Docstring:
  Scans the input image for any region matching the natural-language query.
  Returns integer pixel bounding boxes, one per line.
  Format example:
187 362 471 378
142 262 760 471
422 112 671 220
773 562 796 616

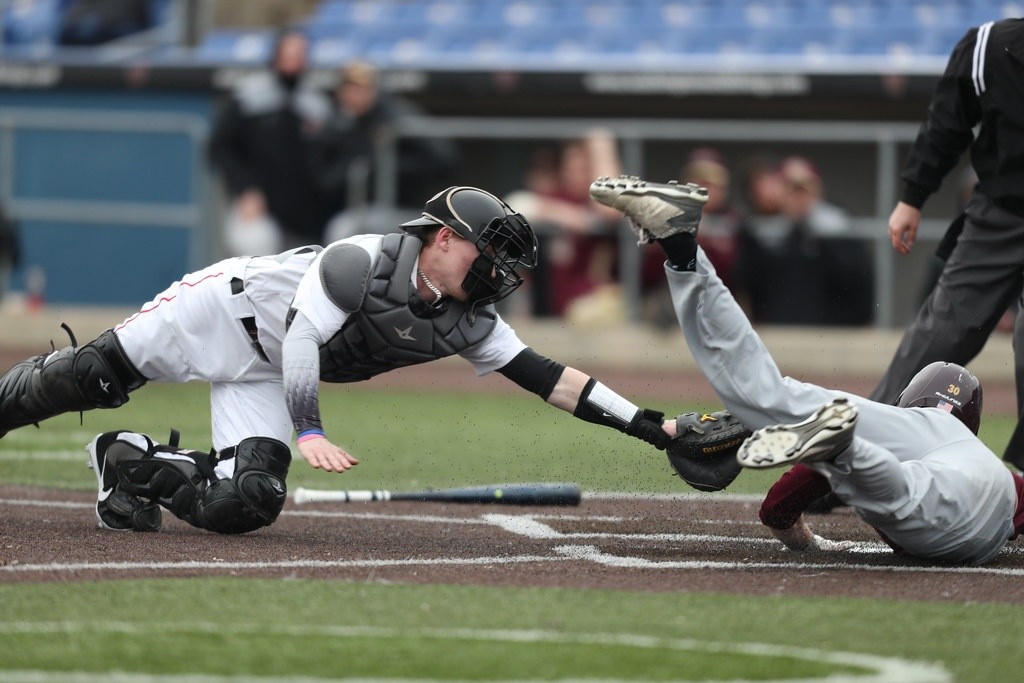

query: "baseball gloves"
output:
663 408 757 496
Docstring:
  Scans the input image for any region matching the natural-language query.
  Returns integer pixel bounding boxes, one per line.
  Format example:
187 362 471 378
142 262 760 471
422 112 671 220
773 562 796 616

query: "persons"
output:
198 30 380 255
592 174 1024 557
492 130 873 325
2 185 747 540
868 17 1023 471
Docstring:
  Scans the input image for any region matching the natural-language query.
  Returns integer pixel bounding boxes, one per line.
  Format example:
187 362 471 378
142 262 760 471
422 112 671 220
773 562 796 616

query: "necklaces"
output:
418 268 446 308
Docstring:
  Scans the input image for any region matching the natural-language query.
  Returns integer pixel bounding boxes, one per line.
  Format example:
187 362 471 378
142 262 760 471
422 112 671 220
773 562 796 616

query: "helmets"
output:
898 362 983 440
400 185 538 271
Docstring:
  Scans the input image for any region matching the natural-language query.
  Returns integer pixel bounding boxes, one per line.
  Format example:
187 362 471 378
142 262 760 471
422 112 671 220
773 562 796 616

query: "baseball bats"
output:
295 479 583 508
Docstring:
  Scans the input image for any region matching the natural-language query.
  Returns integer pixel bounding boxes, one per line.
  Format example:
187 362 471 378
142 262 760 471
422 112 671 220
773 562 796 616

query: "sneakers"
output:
738 398 859 470
590 175 710 243
86 430 143 532
